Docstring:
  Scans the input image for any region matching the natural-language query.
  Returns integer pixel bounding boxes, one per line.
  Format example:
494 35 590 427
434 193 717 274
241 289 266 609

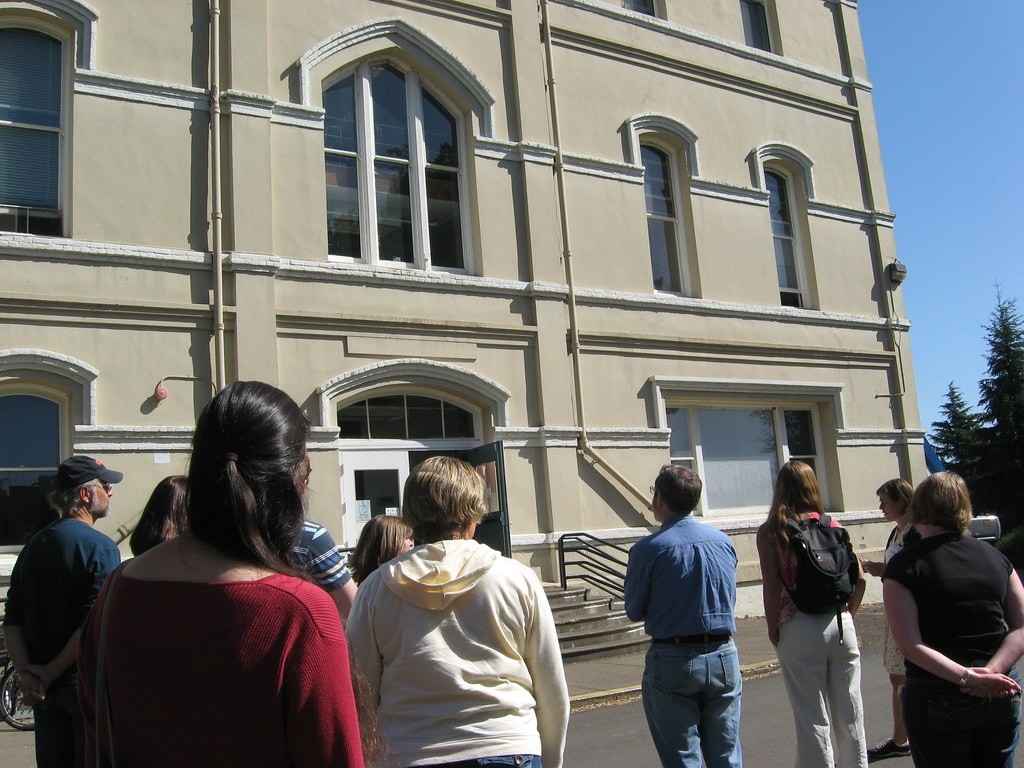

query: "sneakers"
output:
868 737 911 757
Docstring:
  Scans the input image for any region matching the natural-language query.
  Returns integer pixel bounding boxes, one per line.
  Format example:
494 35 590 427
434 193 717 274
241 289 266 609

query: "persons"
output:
856 477 916 758
349 514 415 588
2 454 125 767
76 381 369 768
343 456 571 768
879 471 1024 768
755 460 868 768
624 465 744 768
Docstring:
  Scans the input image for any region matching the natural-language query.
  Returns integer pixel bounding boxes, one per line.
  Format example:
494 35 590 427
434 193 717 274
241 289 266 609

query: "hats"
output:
58 456 123 488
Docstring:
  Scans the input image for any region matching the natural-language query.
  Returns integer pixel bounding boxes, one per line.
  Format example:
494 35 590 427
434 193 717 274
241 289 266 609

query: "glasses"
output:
90 485 112 493
649 486 658 494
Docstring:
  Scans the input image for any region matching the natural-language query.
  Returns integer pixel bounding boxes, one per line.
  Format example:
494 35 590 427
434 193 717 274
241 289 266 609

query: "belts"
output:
653 634 729 644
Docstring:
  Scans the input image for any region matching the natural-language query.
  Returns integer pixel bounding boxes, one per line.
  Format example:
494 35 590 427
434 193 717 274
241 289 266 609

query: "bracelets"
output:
958 670 975 688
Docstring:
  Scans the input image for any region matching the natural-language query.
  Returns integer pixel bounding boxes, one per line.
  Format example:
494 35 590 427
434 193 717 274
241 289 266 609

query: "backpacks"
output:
779 514 860 644
